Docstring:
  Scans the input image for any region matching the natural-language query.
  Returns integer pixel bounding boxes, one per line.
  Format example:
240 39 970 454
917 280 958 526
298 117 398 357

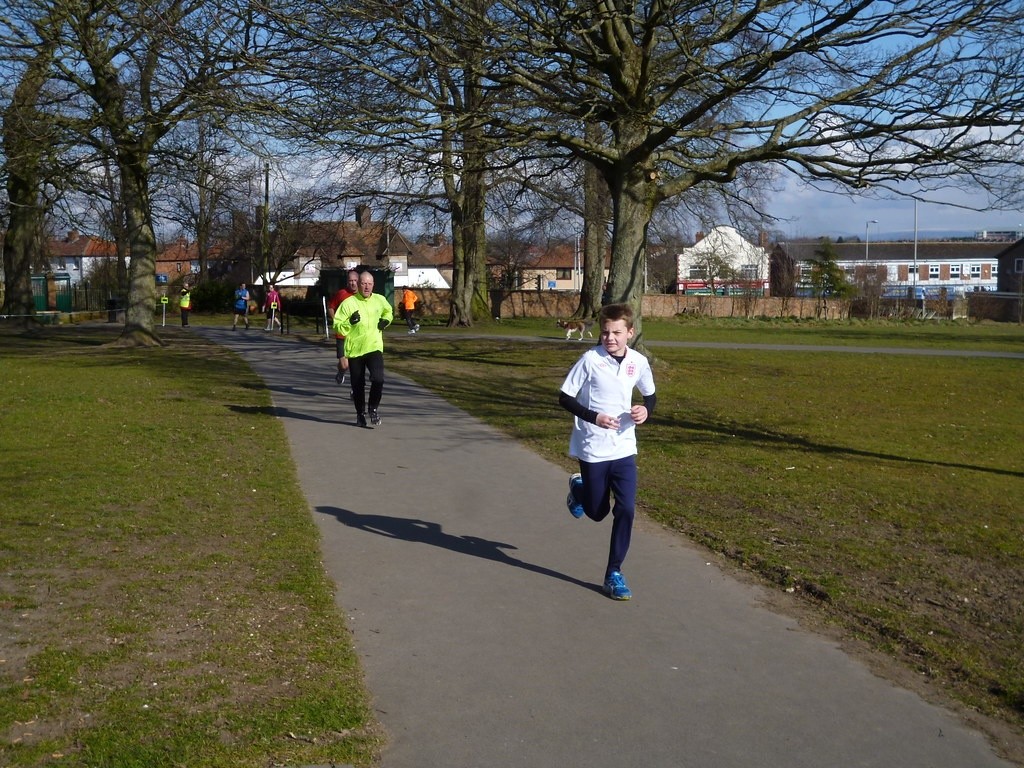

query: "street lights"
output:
865 220 879 266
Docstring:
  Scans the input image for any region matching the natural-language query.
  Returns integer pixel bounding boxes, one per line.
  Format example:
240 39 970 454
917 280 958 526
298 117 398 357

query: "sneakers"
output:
355 412 367 428
566 472 584 518
369 409 382 425
602 571 633 600
335 372 346 383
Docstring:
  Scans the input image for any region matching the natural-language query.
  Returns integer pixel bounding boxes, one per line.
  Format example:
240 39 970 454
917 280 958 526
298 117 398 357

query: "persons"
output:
179 283 192 327
263 283 282 331
231 282 250 331
333 271 393 427
401 286 420 334
328 270 358 399
559 303 657 601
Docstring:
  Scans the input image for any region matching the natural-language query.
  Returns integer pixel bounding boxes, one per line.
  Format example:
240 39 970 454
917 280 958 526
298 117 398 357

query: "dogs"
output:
556 319 592 341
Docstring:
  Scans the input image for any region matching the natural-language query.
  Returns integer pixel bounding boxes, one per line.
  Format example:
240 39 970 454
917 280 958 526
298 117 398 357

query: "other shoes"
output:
232 325 236 331
414 324 420 332
408 329 415 334
244 326 251 332
264 326 271 331
278 324 284 330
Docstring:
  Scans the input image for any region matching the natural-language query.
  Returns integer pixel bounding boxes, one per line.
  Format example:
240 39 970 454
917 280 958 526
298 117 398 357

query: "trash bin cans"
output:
106 299 126 324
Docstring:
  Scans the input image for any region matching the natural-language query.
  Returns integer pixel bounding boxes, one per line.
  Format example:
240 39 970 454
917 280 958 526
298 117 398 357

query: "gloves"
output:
350 310 360 325
378 318 390 331
